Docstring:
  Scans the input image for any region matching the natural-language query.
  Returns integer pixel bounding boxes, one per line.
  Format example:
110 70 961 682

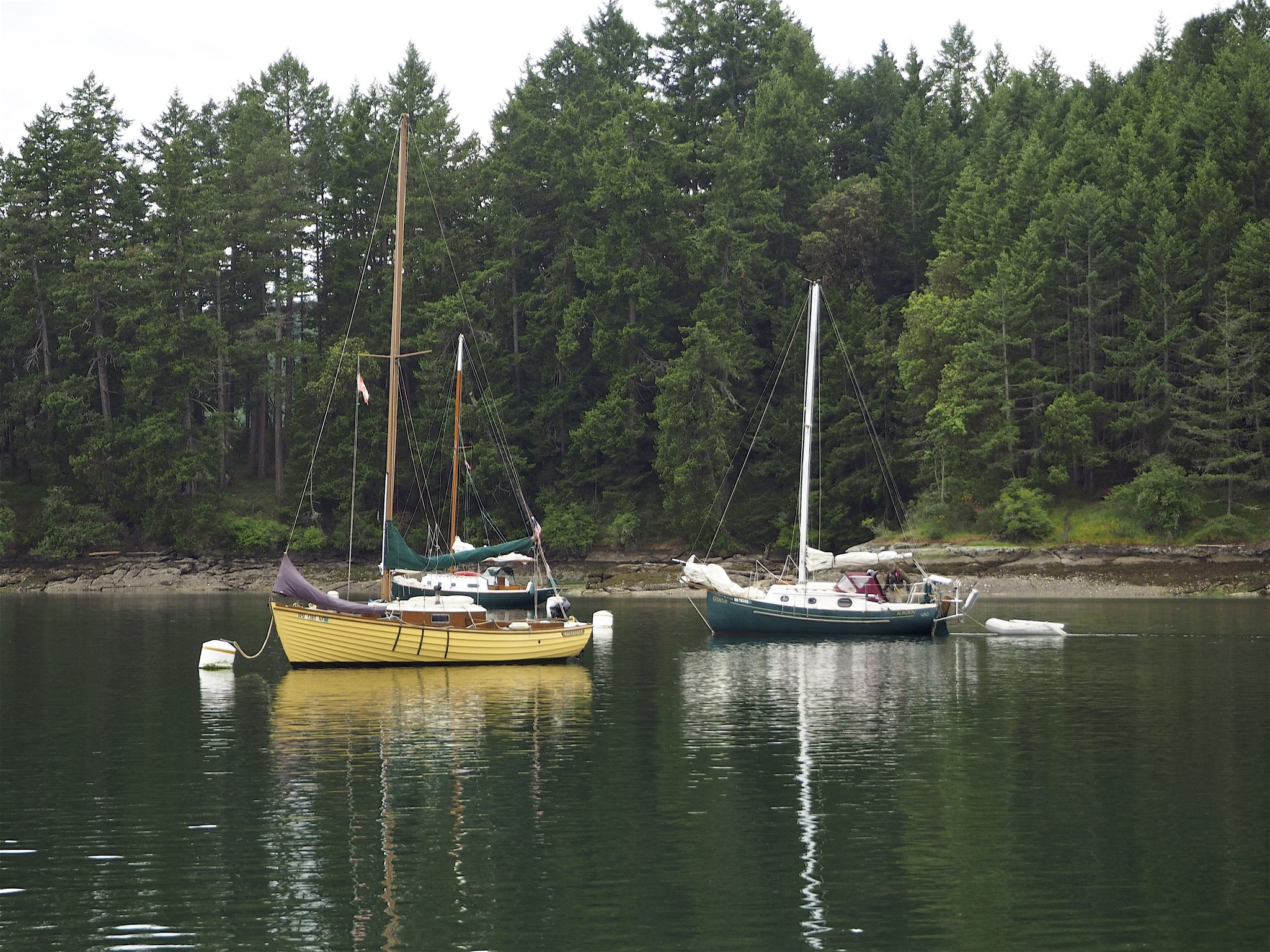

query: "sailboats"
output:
664 276 982 638
268 112 594 670
394 332 566 610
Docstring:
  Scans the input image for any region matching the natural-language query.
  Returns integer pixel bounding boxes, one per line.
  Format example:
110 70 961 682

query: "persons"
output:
882 561 910 603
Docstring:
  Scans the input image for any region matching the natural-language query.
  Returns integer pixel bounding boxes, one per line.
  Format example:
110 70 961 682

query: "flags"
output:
532 516 541 544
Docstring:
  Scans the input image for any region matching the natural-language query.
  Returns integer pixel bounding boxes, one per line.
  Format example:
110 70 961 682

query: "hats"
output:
867 569 878 576
